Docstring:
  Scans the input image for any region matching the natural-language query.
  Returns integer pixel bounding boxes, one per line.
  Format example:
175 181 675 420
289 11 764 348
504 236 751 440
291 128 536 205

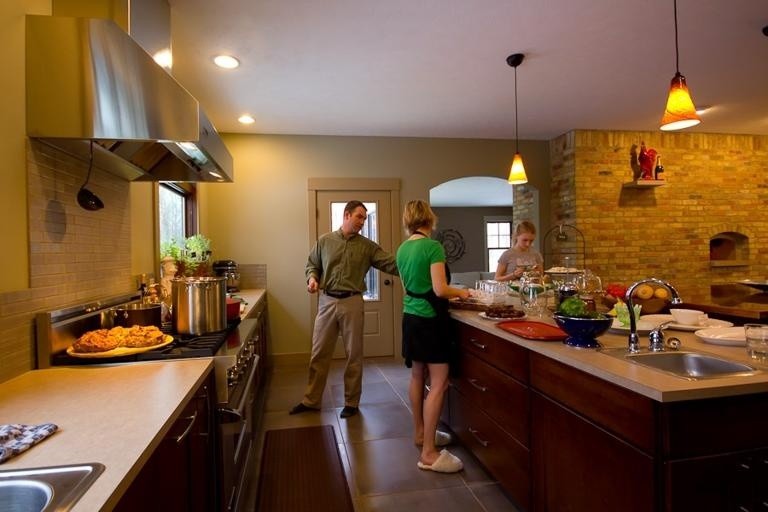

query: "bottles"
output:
139 273 161 303
655 154 665 181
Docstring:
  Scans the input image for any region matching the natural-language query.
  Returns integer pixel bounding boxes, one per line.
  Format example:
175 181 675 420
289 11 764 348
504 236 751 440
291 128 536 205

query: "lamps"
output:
657 0 703 132
505 51 528 185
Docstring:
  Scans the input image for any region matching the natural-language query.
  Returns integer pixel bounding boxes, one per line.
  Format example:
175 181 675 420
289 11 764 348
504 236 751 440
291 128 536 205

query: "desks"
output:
695 301 767 322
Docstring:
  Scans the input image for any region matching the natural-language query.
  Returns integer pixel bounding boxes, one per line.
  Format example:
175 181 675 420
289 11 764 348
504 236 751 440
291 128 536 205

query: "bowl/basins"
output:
668 308 704 326
225 299 242 321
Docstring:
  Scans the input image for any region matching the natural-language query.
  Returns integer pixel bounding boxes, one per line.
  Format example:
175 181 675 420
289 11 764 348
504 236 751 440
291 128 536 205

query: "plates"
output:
694 327 745 346
607 315 652 336
477 310 528 320
639 313 734 333
66 329 174 359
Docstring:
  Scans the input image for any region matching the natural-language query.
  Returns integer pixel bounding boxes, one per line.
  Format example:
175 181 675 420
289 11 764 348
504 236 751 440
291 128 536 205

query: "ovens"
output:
216 354 260 512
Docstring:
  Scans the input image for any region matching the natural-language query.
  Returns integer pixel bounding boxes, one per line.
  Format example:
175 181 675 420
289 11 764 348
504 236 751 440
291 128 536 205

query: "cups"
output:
743 324 766 369
474 279 510 299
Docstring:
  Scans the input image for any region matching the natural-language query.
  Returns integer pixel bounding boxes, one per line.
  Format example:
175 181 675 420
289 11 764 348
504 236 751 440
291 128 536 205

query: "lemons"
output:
637 286 654 299
654 288 668 297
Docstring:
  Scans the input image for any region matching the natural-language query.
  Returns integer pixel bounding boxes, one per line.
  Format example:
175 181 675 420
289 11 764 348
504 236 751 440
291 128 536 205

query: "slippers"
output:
434 429 452 446
417 449 465 474
340 406 359 419
288 403 315 416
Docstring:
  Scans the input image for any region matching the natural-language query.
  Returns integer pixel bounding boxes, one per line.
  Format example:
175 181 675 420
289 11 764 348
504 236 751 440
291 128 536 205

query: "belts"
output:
324 291 361 298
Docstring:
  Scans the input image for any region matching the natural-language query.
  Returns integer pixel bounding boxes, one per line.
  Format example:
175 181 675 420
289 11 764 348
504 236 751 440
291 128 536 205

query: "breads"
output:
72 324 166 353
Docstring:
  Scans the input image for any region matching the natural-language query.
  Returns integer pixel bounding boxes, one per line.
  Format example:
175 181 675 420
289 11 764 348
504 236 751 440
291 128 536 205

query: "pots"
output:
168 276 227 336
110 303 160 326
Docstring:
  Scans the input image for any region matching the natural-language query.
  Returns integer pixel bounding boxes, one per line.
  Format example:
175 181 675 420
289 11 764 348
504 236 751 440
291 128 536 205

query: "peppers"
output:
560 296 586 316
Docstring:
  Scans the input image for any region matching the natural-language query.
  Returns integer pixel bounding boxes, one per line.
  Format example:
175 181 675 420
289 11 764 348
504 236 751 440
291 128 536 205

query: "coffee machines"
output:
212 260 240 292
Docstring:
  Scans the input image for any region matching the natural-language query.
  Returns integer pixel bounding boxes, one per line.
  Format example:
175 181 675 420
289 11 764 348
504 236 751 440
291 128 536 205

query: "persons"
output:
494 222 544 284
395 200 473 474
290 200 399 418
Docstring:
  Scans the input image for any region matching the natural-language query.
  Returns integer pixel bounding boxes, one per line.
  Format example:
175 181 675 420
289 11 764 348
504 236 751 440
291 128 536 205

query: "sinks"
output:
622 348 758 380
0 461 106 512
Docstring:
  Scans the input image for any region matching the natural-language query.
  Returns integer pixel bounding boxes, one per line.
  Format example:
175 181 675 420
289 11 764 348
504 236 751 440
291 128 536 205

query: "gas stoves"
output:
51 318 261 407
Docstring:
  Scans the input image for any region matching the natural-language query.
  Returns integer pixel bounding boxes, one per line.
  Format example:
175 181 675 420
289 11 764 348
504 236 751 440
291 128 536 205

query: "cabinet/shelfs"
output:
247 292 271 443
422 363 450 432
112 367 222 512
450 317 527 512
528 346 766 512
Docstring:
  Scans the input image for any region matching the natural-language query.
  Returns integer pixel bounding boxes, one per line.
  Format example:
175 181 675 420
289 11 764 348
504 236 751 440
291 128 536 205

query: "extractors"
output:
26 0 235 182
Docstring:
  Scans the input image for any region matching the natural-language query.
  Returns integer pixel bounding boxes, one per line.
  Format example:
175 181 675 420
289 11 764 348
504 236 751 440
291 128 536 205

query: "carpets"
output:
251 424 354 512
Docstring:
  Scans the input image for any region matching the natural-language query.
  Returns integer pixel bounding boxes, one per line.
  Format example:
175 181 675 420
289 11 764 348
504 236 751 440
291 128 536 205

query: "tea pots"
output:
516 266 558 315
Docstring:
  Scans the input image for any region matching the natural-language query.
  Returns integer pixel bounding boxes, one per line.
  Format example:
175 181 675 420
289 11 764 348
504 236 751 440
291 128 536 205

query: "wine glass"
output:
553 312 616 350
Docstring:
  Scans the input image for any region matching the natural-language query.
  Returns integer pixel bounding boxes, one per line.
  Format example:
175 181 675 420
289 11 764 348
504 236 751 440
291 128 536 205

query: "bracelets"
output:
512 273 516 279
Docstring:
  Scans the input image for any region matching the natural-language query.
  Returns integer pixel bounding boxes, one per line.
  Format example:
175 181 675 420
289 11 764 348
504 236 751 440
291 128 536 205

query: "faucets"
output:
623 275 685 353
